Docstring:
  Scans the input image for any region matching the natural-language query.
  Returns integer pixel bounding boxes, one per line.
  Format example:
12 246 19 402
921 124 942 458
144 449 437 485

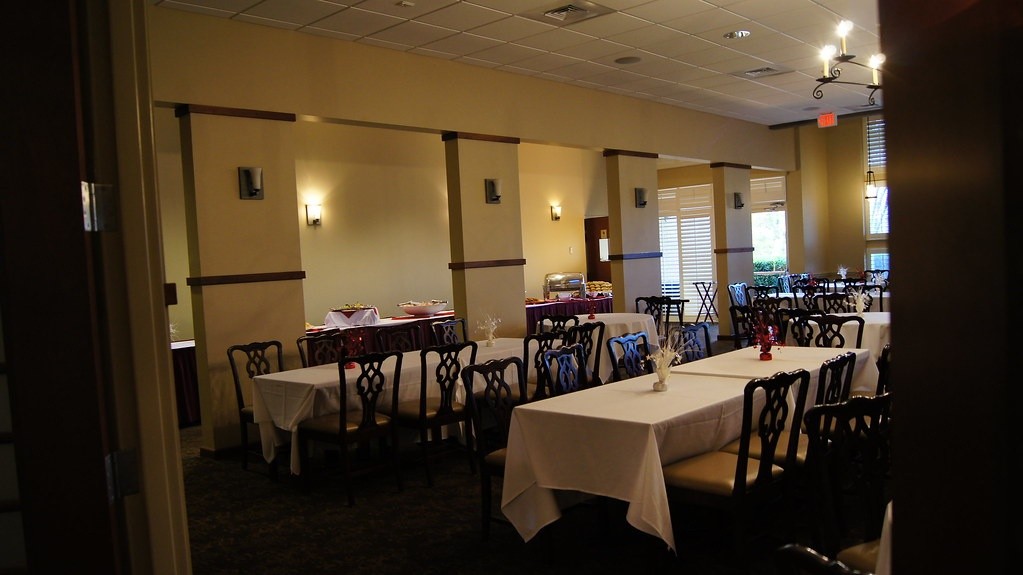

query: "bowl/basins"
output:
403 304 447 316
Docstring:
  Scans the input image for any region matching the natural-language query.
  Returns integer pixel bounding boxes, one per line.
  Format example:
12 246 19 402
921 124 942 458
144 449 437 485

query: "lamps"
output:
492 178 502 201
639 187 649 205
248 167 262 196
551 206 561 221
865 112 877 200
311 203 320 223
738 193 744 207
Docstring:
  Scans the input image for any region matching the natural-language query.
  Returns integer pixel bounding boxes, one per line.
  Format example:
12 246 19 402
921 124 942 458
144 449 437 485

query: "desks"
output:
458 336 578 403
251 350 457 477
788 311 889 362
538 313 659 383
668 343 878 434
512 371 766 575
754 292 844 311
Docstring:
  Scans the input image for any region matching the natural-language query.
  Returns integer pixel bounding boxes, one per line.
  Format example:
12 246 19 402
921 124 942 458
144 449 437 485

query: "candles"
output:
838 21 847 54
873 56 878 85
824 47 829 77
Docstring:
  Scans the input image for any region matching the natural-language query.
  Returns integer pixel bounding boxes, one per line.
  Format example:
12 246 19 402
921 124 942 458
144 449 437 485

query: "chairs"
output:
228 270 889 575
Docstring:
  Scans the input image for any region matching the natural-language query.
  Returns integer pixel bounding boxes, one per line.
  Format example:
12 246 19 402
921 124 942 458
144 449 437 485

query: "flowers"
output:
837 263 848 274
639 337 699 366
850 288 867 307
753 323 784 351
473 312 501 335
584 297 598 309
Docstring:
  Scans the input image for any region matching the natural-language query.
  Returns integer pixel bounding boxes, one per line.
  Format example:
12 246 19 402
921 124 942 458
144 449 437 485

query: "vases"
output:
486 336 495 347
760 345 772 360
653 368 668 392
841 275 847 279
588 308 596 319
856 308 865 317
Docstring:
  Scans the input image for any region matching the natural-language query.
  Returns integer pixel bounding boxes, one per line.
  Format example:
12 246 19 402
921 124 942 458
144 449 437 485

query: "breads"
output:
585 282 612 291
